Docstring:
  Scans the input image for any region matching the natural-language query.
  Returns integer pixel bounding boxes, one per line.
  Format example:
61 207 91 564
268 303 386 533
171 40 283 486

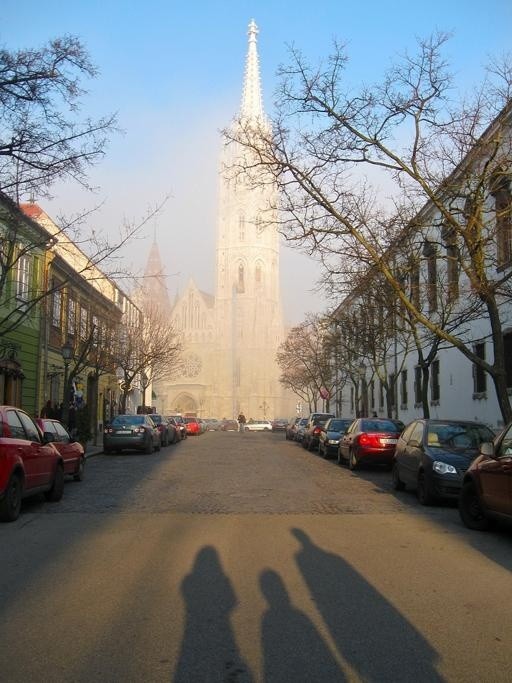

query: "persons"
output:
237 411 246 433
40 401 91 451
372 411 377 417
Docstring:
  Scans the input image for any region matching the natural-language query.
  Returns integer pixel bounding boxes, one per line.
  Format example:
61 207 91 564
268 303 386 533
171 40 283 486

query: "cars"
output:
0 405 65 524
462 420 512 530
244 419 287 431
205 418 238 431
392 418 496 505
103 413 161 454
288 412 355 458
149 414 204 447
337 417 405 469
37 418 85 481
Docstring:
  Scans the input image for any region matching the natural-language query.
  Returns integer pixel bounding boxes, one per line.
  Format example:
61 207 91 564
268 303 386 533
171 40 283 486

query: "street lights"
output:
62 342 75 423
141 374 148 413
358 362 367 417
